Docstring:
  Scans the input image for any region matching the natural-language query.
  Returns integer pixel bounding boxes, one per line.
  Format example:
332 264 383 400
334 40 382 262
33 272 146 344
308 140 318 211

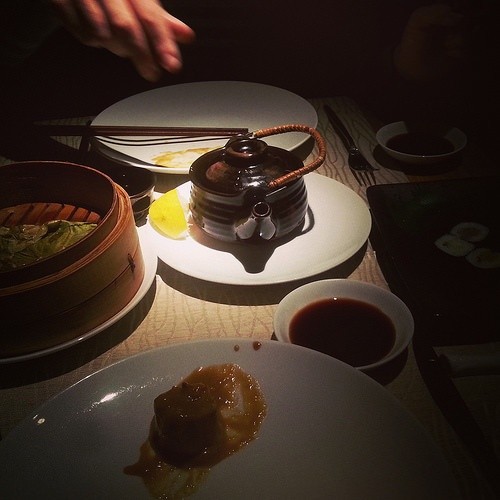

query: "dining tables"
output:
0 96 499 500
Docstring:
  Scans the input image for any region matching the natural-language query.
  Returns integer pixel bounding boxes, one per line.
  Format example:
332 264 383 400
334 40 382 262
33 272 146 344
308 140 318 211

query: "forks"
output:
324 104 380 187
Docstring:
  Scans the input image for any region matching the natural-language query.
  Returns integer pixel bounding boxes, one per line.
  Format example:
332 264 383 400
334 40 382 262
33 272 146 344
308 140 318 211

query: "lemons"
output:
149 189 189 239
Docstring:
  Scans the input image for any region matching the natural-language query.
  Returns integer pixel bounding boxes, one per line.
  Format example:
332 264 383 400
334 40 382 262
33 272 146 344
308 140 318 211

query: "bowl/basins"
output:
94 168 155 202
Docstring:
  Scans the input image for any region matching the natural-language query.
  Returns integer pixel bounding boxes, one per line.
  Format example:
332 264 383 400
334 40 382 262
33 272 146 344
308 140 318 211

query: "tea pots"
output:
189 124 326 242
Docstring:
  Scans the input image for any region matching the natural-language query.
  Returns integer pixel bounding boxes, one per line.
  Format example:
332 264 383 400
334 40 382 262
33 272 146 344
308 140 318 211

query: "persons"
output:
78 0 194 82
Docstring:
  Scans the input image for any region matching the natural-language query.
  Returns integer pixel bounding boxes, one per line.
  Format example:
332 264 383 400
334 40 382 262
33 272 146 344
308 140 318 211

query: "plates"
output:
2 339 456 499
377 122 467 161
145 172 372 285
274 279 414 370
1 225 158 361
366 176 500 342
89 81 318 173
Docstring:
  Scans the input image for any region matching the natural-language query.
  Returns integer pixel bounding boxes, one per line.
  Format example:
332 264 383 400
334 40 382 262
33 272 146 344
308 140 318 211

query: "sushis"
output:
432 220 500 285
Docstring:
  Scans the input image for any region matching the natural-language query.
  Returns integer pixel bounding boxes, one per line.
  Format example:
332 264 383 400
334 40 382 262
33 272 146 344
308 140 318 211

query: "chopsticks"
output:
19 123 249 137
